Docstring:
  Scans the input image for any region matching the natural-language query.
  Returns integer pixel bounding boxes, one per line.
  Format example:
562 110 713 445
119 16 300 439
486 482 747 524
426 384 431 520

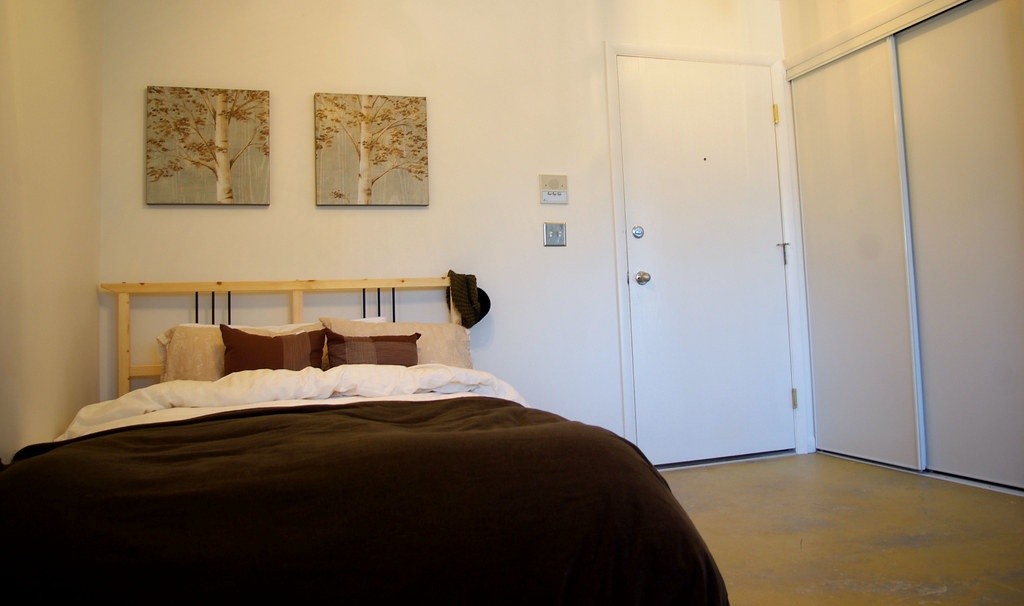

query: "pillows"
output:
156 321 325 383
319 316 473 370
325 327 421 369
220 323 324 375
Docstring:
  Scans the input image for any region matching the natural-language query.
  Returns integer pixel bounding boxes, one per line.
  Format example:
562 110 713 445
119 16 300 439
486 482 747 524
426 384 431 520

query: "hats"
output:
446 271 491 328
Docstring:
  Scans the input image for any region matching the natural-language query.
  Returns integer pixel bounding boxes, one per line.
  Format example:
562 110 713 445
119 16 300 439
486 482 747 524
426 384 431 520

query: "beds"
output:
0 278 730 606
